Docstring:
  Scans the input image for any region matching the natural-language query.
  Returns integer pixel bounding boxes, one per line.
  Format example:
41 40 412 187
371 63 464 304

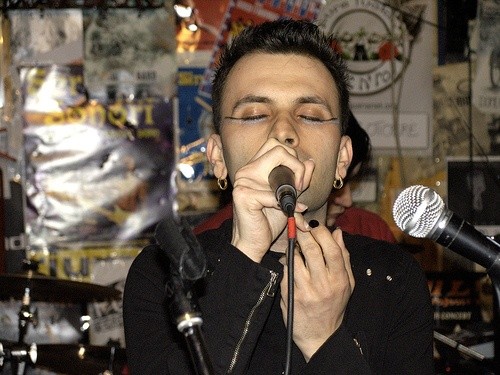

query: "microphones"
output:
392 184 500 282
268 165 297 215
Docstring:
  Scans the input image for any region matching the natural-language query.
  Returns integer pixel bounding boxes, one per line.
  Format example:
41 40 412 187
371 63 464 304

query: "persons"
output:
193 106 399 246
123 15 435 375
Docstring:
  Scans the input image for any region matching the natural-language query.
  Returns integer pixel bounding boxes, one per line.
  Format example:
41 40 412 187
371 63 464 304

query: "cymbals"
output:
0 273 122 301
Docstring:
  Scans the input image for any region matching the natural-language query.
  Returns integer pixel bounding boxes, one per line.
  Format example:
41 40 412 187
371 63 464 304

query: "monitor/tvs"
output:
444 156 500 237
